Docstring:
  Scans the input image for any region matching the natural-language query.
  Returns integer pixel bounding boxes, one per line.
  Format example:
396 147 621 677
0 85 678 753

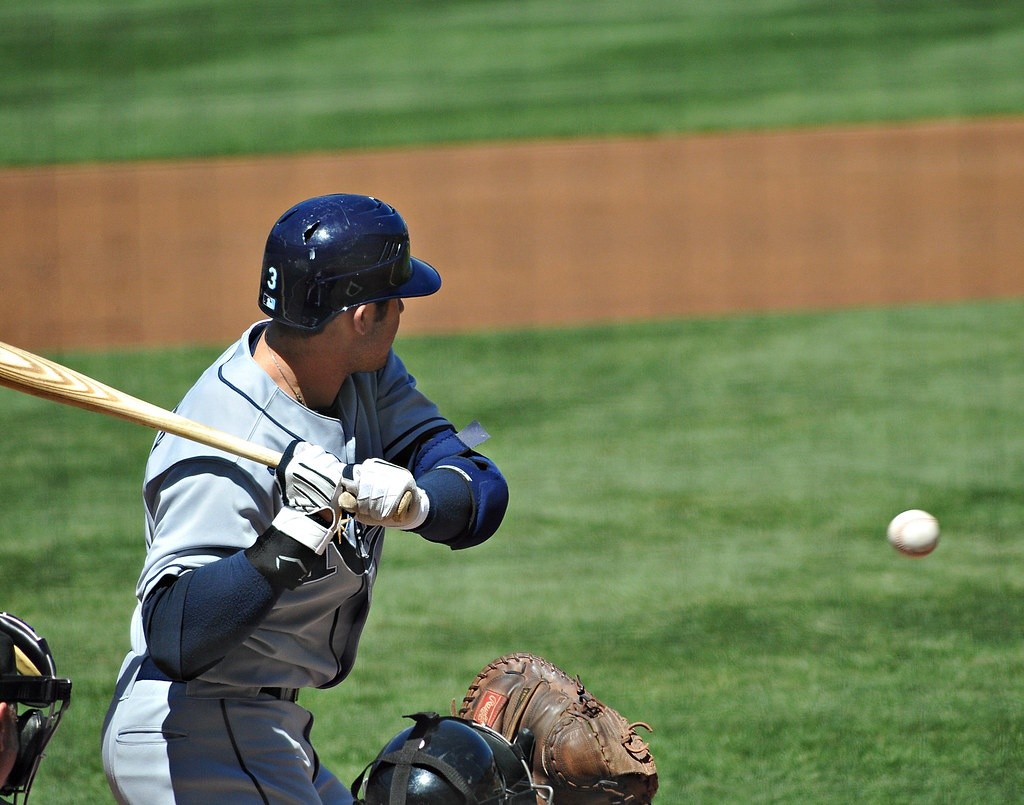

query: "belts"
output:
258 685 301 703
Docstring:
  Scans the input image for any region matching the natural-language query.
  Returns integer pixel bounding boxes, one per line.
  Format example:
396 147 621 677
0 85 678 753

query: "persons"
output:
101 193 509 805
0 611 72 805
351 711 554 805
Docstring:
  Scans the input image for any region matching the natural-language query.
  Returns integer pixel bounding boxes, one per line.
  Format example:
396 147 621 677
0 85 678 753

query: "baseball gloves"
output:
458 655 660 804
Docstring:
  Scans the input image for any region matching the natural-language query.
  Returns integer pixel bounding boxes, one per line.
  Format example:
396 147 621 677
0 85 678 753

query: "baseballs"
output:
887 510 938 559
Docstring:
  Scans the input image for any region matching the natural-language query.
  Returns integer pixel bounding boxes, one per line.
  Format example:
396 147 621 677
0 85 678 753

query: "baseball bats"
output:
0 342 414 520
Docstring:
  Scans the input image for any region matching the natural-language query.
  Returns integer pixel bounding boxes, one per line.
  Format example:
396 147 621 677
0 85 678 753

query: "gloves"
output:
270 437 343 555
338 457 431 532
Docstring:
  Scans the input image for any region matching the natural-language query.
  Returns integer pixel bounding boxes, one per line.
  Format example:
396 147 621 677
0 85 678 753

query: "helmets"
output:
348 710 540 805
257 189 444 329
